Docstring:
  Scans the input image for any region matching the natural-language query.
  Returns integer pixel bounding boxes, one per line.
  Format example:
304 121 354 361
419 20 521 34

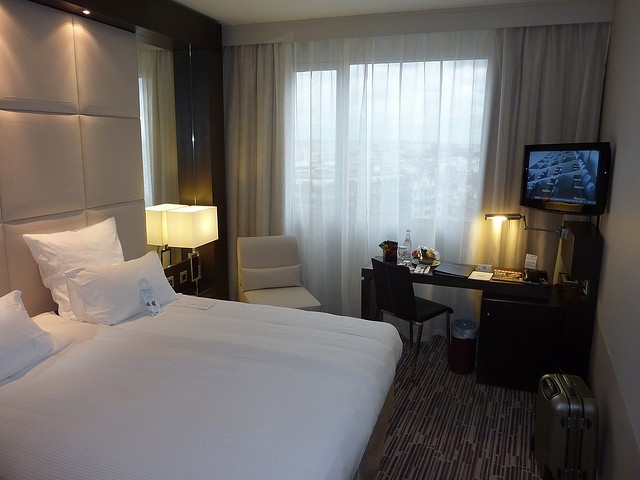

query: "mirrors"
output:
135 25 195 268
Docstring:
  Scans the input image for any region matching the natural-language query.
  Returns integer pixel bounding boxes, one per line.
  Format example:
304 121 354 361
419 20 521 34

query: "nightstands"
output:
164 258 228 299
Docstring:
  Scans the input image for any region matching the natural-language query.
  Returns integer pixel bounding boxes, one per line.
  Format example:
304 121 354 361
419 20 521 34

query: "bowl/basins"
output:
412 258 441 273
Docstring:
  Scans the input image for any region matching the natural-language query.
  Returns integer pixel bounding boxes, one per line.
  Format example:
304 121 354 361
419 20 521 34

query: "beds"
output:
1 293 405 480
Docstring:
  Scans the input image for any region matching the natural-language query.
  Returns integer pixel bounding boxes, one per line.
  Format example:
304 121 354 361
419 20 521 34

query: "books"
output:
433 262 474 279
468 271 493 281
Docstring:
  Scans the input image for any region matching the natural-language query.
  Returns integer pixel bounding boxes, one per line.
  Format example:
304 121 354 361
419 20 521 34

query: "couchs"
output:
237 234 321 311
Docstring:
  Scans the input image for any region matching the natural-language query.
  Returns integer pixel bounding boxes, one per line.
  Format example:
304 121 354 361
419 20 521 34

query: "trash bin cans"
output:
448 319 477 375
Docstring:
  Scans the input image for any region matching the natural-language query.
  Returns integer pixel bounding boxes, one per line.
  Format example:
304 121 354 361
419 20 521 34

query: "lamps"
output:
166 206 219 294
146 203 188 267
484 213 570 240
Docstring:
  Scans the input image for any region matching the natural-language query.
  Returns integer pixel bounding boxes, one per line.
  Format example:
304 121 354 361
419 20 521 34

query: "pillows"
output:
1 289 75 383
239 265 302 292
22 217 126 319
65 250 183 325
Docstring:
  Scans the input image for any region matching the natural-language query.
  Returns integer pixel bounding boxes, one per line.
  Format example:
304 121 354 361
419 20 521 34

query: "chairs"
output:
370 257 454 382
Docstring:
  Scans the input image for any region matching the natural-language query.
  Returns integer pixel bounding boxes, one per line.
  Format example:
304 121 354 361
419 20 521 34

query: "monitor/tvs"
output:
518 141 613 216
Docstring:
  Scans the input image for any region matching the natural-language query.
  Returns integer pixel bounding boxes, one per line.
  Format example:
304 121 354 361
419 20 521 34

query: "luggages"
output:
531 373 599 479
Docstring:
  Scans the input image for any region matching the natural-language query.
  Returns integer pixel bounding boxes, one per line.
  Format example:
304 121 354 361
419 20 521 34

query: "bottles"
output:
404 230 412 260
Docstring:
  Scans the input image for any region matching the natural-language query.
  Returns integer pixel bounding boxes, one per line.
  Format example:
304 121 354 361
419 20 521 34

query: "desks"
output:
359 221 605 392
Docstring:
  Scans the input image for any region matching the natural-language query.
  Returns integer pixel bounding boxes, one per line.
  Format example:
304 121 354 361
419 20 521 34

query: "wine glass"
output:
398 246 408 264
402 256 411 266
